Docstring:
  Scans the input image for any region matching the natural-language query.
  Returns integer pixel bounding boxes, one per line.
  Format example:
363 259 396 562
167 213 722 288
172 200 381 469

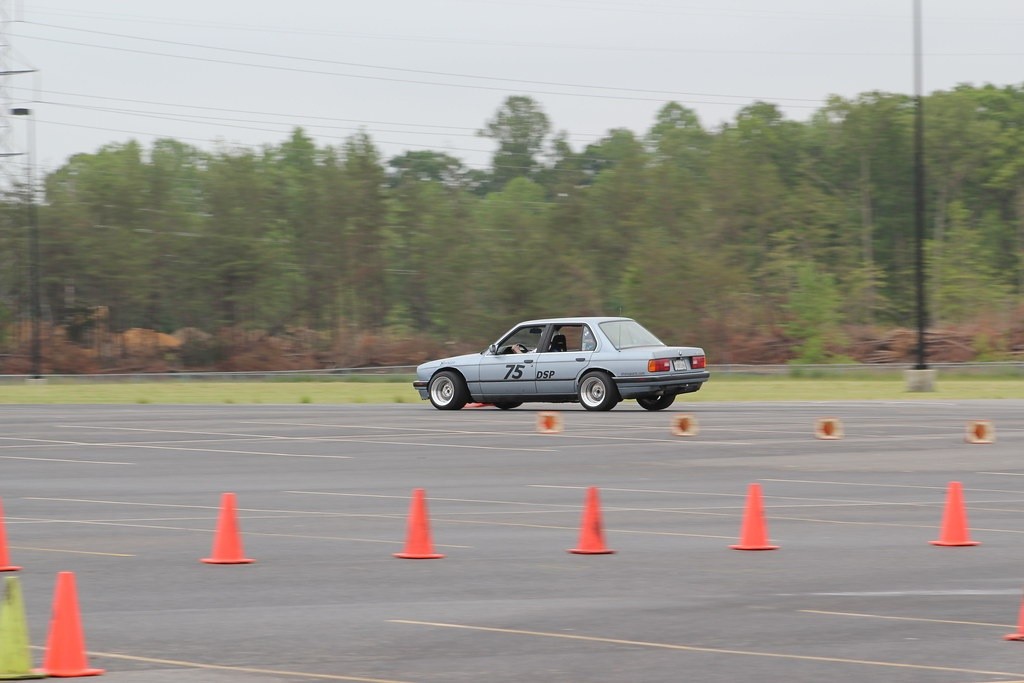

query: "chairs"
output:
550 334 567 351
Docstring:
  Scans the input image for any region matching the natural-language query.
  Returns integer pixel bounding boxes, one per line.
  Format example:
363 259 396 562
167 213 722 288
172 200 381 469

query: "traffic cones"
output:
1 574 47 679
729 479 779 551
536 409 563 436
0 495 24 573
36 572 107 677
813 414 843 441
927 480 982 546
566 484 620 555
672 414 698 436
389 485 445 560
200 491 256 564
1006 590 1024 640
965 418 996 445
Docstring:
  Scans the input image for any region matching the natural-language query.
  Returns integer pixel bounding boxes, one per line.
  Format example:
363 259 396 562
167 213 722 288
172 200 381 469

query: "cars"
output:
412 316 711 411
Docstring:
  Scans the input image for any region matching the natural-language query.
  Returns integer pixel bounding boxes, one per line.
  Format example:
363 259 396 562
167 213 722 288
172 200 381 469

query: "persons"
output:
511 330 560 354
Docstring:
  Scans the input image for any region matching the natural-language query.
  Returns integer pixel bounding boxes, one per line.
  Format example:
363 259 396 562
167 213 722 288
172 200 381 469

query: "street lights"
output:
8 106 43 383
